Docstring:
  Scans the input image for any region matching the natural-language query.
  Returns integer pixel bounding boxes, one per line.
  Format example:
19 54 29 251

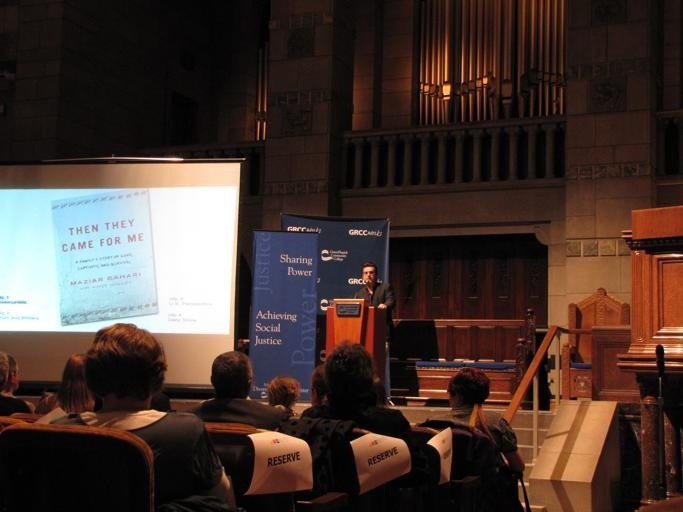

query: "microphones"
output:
365 283 373 295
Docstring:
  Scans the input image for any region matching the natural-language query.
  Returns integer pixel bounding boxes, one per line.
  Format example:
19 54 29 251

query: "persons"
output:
353 262 396 408
0 321 524 512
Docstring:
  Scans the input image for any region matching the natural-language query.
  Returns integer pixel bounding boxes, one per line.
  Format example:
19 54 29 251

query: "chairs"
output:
560 286 629 402
0 412 526 512
